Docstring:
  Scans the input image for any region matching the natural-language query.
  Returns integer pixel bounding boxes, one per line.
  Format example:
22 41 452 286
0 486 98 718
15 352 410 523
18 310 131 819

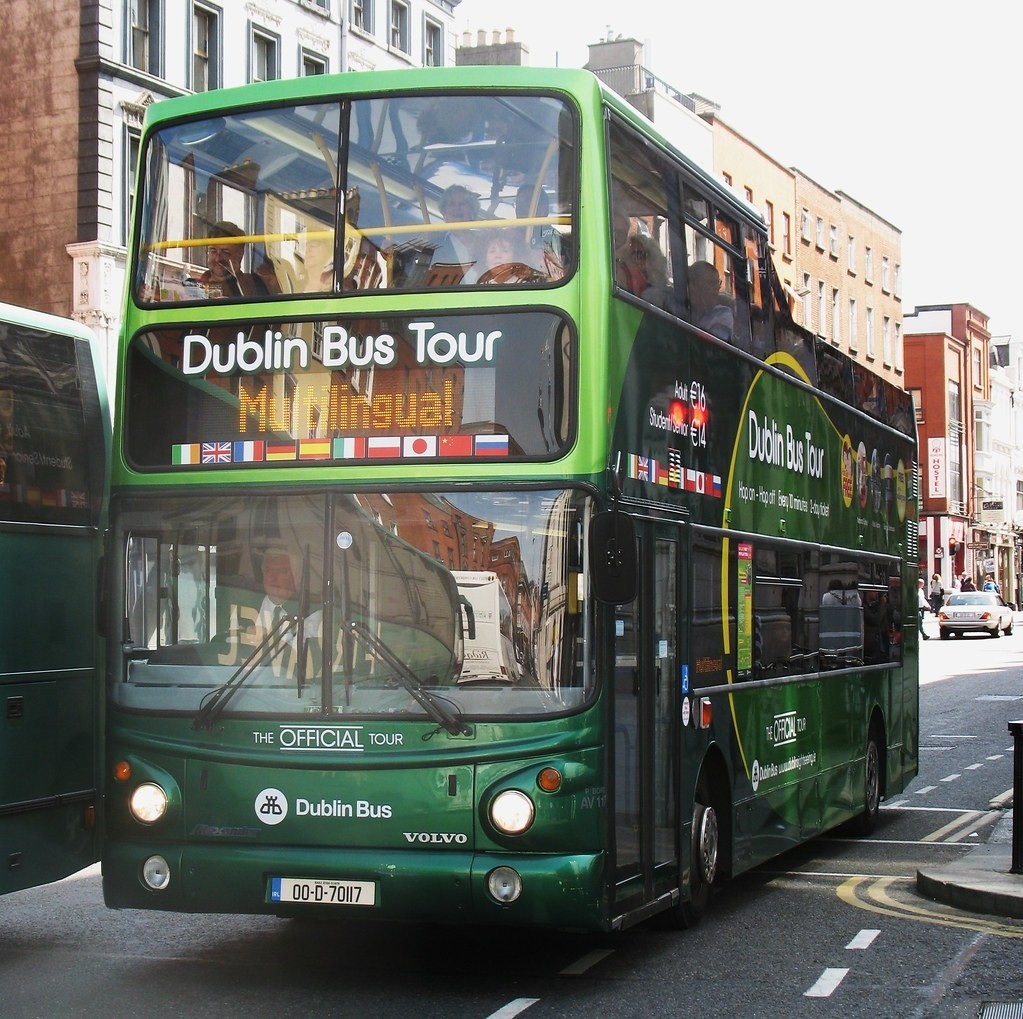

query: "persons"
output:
296 223 372 293
867 590 899 660
982 574 999 593
232 541 318 667
917 578 932 640
930 573 943 617
960 571 975 591
183 218 272 298
613 203 768 359
427 178 571 286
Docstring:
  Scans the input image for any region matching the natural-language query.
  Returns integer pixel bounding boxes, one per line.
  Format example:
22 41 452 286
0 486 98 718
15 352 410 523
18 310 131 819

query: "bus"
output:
532 491 589 708
94 66 920 936
0 300 114 895
451 569 521 684
123 334 475 688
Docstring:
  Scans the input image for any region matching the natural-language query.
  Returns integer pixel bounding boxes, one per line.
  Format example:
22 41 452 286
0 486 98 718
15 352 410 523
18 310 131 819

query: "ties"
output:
272 606 282 659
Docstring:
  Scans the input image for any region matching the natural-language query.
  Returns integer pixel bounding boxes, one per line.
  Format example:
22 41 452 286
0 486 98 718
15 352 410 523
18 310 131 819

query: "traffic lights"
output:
949 538 956 556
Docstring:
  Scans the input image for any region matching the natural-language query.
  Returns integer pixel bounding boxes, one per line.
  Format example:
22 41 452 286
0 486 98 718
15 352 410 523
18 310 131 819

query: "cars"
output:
939 591 1017 639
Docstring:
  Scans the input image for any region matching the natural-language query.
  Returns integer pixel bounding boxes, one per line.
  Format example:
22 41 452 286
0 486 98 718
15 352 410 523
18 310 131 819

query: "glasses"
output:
207 248 239 258
697 278 722 286
632 250 646 260
